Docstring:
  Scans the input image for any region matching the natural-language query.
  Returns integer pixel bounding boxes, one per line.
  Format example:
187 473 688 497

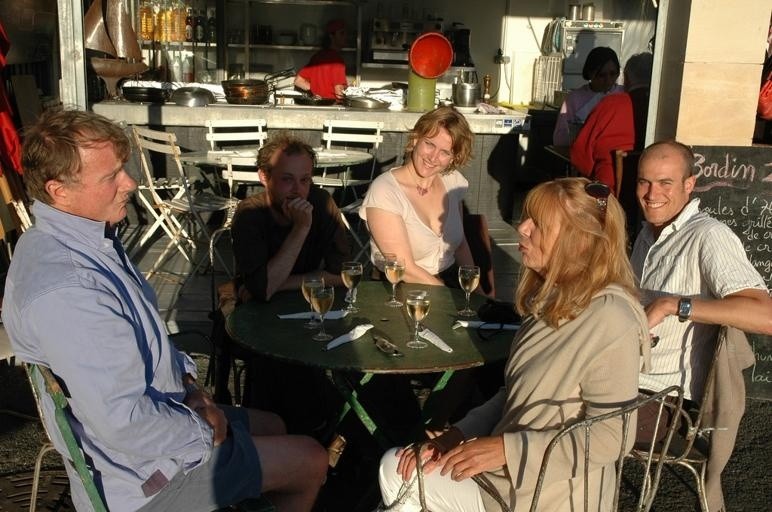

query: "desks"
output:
226 279 524 442
206 146 374 189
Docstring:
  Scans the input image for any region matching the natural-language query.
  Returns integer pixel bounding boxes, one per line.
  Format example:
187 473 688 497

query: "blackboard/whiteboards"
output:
689 145 772 401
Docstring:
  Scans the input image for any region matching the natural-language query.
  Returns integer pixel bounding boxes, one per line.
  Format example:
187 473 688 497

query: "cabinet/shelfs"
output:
129 1 365 96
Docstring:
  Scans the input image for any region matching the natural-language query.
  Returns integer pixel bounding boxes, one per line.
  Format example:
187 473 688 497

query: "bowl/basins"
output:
171 86 217 108
409 33 454 79
451 81 481 106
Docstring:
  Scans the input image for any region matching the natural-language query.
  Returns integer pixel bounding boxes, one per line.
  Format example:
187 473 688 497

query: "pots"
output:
222 67 298 104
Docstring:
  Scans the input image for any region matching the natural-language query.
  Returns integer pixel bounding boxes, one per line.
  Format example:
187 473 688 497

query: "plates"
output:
453 104 477 113
292 94 392 110
119 85 168 103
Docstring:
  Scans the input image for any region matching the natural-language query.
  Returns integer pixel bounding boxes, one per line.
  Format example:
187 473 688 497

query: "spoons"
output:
375 336 404 359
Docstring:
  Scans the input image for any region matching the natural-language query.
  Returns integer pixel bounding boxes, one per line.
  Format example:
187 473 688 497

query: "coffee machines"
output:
367 18 443 61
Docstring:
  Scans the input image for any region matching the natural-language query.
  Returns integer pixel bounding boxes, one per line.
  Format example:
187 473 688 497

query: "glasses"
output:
584 176 610 229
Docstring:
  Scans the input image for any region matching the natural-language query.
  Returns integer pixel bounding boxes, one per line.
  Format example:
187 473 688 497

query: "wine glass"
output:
405 289 431 349
384 257 407 309
457 263 481 318
301 274 325 329
310 284 336 342
341 261 364 313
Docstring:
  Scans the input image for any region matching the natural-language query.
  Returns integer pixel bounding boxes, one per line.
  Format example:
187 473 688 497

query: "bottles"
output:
582 3 596 20
171 55 182 83
569 4 581 21
182 57 193 83
376 2 439 20
482 73 492 104
137 0 215 42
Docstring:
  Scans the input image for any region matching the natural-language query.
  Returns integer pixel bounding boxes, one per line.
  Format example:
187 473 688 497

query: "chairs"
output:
204 120 269 196
25 363 108 511
203 227 248 406
220 157 261 199
132 124 242 279
317 120 384 189
336 199 372 268
619 322 731 509
414 384 685 511
